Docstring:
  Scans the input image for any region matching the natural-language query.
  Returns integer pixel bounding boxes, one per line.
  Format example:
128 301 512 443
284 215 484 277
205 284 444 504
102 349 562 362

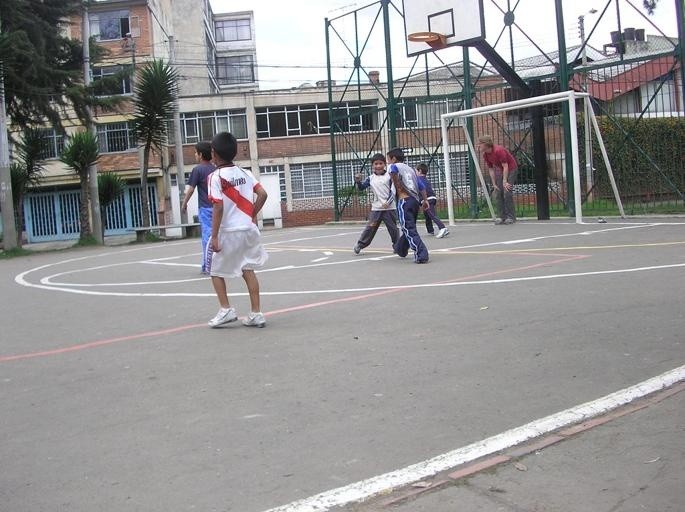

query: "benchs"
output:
126 223 200 242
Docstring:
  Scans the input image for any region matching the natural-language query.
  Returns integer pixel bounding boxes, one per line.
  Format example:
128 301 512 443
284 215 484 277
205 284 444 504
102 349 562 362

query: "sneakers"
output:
242 312 266 326
436 228 450 239
495 219 514 224
354 243 361 255
208 307 237 327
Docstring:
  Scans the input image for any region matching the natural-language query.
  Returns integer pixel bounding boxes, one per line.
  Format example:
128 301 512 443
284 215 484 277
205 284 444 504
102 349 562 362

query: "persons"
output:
413 165 450 239
476 134 517 224
389 150 431 264
353 153 400 257
179 140 219 274
202 132 270 327
